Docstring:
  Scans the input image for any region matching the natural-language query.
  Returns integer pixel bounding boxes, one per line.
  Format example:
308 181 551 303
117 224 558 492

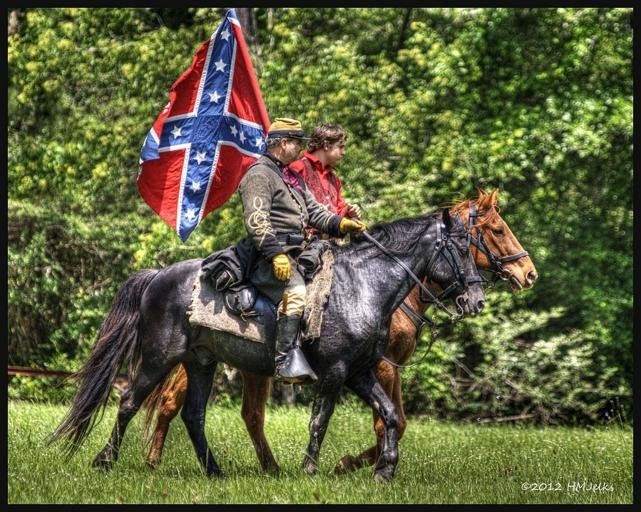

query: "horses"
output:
43 207 486 485
143 185 540 474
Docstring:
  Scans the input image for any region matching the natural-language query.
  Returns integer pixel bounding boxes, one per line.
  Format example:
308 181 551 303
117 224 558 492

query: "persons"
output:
237 118 366 384
290 125 363 245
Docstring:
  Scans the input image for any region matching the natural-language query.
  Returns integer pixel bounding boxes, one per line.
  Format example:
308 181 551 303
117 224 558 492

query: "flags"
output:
136 8 270 243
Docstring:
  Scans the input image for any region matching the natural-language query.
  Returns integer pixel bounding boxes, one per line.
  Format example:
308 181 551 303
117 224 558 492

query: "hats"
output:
268 118 313 141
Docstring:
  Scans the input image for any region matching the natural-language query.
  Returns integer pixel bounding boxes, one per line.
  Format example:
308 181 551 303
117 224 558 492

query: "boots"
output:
272 315 306 384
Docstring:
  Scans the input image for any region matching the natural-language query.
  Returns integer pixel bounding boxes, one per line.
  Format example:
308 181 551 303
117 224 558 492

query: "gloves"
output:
340 217 366 237
273 253 292 281
